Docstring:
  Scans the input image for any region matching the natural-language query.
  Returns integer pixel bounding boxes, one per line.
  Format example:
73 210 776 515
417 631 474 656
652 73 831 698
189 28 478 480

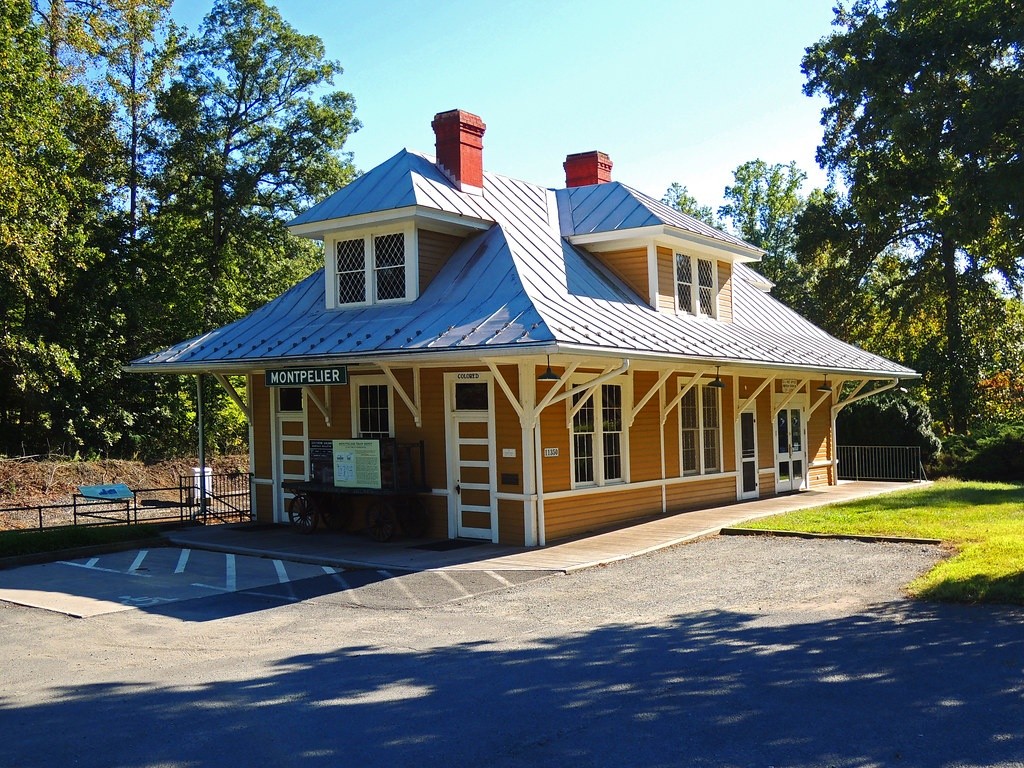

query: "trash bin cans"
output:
188 468 212 499
